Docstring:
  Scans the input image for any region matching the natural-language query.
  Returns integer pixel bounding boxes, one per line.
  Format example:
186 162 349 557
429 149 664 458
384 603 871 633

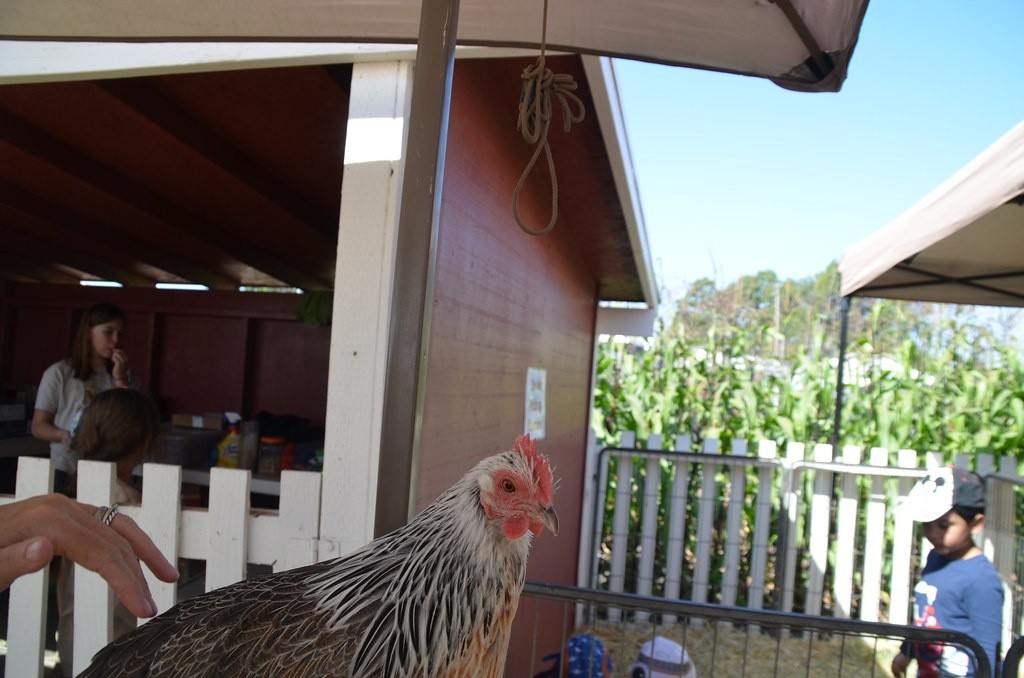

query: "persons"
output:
0 306 182 622
891 465 1006 678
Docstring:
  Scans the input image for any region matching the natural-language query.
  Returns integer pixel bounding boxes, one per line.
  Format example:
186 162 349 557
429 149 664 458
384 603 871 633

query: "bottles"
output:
218 414 295 480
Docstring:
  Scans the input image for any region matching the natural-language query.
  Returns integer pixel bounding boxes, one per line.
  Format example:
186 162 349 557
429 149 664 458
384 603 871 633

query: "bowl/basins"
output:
158 433 223 467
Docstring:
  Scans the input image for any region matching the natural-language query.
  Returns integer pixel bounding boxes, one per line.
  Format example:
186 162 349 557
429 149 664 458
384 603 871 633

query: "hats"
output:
892 465 985 523
631 636 697 678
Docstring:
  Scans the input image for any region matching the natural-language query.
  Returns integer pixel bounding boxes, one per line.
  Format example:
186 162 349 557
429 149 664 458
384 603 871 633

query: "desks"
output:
163 426 227 468
133 464 280 496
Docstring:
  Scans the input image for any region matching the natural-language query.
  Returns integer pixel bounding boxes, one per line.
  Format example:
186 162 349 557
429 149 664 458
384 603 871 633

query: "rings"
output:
95 503 120 525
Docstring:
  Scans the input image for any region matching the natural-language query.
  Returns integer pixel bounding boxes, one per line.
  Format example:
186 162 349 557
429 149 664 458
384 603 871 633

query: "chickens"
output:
75 433 559 677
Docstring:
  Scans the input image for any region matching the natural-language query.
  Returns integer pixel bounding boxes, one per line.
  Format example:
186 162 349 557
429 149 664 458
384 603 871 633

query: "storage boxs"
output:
172 414 223 429
